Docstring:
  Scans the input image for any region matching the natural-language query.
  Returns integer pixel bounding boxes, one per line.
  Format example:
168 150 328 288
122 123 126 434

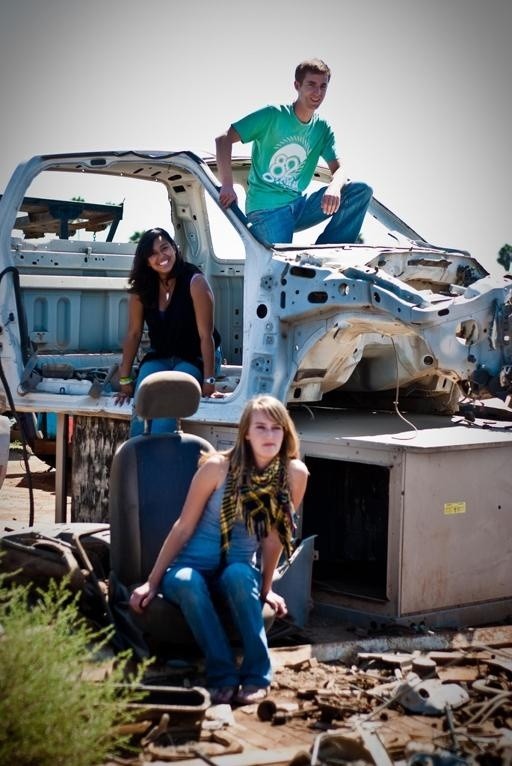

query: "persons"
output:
214 57 374 251
115 227 225 405
130 395 311 702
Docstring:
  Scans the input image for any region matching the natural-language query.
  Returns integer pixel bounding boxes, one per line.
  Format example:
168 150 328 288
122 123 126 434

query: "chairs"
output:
108 366 298 661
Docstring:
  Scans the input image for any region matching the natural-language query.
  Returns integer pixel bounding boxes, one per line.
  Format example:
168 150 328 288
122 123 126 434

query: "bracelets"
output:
117 377 134 385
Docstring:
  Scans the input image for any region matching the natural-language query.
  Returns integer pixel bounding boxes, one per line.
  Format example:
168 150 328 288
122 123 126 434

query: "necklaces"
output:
160 279 176 301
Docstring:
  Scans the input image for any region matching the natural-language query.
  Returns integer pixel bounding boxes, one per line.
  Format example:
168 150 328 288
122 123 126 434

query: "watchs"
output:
204 377 217 385
